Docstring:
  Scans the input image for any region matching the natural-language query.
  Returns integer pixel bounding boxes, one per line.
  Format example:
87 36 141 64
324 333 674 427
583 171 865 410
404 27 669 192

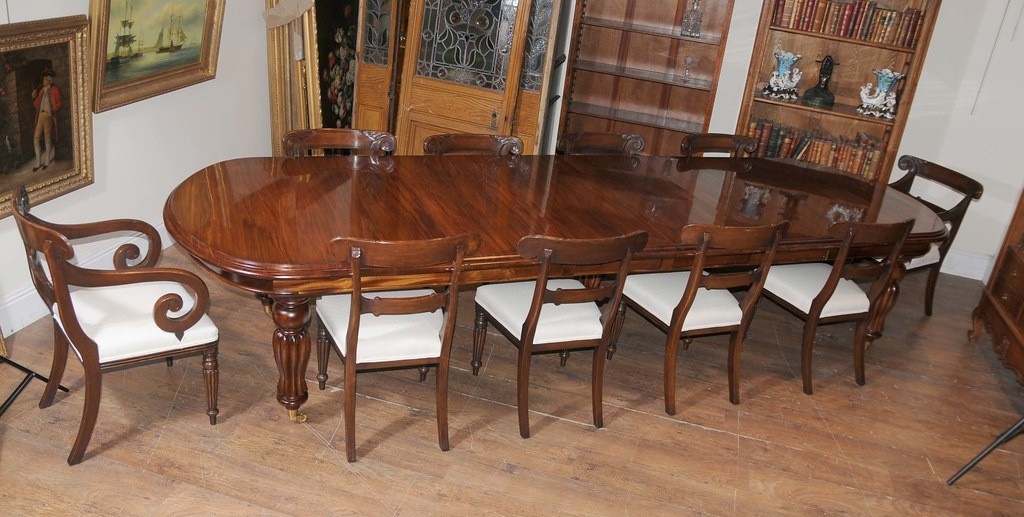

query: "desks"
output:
165 154 950 423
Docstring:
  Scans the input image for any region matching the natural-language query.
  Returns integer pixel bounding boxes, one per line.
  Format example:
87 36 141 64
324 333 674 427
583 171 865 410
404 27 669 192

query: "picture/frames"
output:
0 14 95 220
87 0 226 114
261 0 359 156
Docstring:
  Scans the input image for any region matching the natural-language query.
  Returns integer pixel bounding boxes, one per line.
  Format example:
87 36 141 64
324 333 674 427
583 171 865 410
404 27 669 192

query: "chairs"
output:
12 128 985 466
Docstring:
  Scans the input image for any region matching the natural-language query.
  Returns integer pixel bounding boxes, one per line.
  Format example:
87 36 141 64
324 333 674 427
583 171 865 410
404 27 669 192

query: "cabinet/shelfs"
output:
967 189 1024 387
554 0 735 157
730 0 942 185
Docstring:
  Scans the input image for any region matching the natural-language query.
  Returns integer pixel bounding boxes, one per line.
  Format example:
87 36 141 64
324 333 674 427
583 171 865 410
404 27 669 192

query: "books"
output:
742 115 888 182
771 0 925 50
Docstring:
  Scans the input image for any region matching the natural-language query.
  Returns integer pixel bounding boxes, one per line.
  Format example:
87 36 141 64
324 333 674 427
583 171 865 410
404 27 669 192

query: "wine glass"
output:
682 55 695 80
